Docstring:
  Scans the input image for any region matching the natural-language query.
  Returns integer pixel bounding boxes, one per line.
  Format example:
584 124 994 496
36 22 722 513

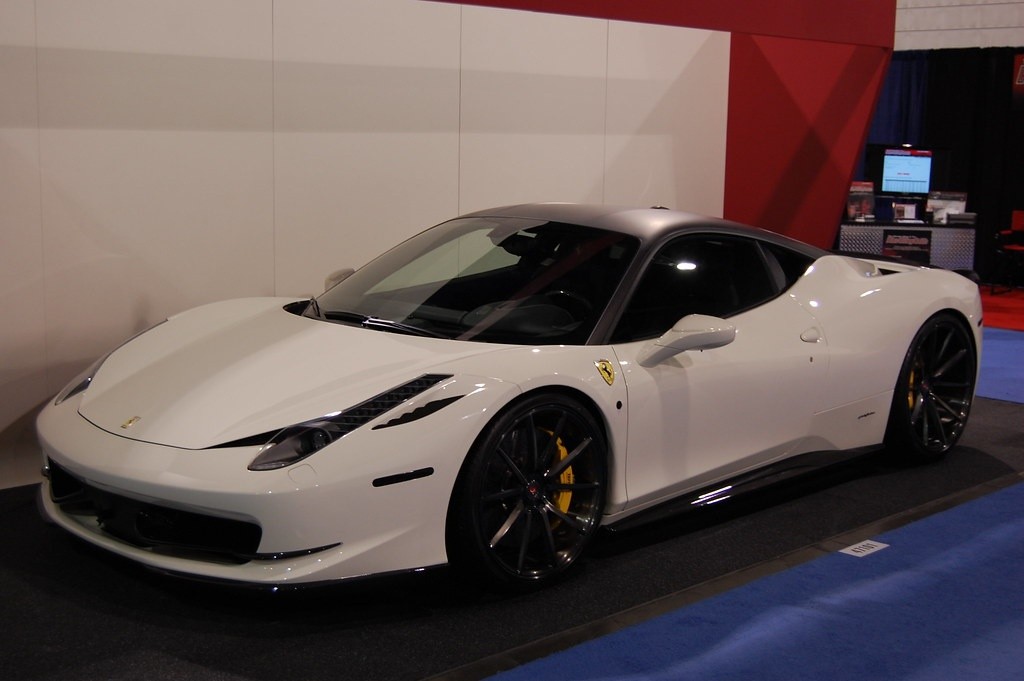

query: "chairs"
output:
991 210 1024 296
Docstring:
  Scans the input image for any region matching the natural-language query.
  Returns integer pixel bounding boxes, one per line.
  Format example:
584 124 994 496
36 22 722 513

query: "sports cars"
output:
34 200 982 603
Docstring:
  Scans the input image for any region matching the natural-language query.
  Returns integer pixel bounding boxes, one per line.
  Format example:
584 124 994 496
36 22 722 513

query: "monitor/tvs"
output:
874 145 938 223
